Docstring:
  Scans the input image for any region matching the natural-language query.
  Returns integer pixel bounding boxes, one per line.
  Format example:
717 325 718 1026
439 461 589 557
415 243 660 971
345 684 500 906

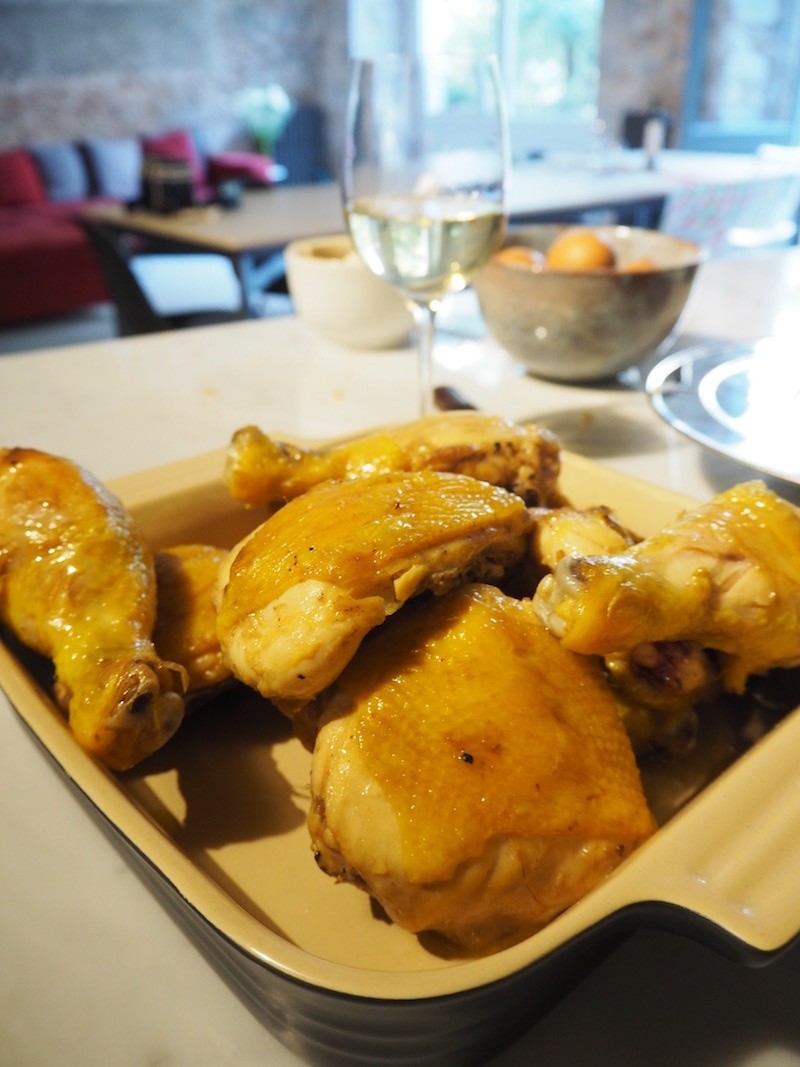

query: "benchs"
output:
0 127 284 327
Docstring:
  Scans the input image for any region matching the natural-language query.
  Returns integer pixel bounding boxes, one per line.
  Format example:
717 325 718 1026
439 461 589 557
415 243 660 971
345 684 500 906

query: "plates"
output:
0 409 799 1066
645 338 800 488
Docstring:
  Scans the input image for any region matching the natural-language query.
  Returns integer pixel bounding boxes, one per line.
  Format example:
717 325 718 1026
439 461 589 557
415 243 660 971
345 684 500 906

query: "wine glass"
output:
341 49 508 417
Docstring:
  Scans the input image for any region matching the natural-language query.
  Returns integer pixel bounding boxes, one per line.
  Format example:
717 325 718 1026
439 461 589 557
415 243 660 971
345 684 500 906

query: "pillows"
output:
78 136 145 203
0 146 46 209
26 137 92 205
145 131 215 201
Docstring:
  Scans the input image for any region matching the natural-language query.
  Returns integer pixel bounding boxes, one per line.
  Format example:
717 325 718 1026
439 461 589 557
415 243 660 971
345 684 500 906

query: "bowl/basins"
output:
283 234 421 347
465 223 707 386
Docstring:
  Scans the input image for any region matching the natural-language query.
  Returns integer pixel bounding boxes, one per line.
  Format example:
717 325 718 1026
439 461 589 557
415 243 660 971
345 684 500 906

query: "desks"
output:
68 144 788 340
0 236 800 1067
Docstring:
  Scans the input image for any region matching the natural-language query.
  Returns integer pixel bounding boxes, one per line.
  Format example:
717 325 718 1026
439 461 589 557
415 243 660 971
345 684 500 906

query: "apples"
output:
497 226 658 272
